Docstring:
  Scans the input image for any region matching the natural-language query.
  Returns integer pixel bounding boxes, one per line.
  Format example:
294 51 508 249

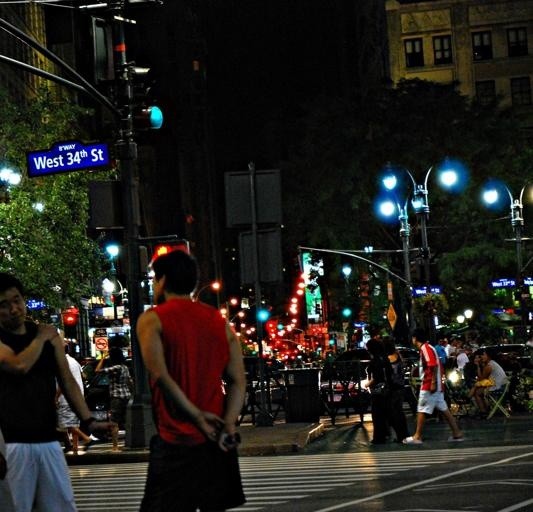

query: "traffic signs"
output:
489 278 515 288
413 287 441 295
30 140 109 175
522 278 533 286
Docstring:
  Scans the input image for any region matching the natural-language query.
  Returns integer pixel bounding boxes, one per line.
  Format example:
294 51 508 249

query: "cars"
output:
223 356 289 410
465 342 533 389
75 360 134 441
317 346 450 414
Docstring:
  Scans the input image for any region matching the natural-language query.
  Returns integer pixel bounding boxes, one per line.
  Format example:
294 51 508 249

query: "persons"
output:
94 346 135 451
56 343 94 455
136 249 247 512
0 273 119 512
364 327 522 445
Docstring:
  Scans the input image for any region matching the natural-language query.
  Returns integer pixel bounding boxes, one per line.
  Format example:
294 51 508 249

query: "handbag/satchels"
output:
474 377 495 386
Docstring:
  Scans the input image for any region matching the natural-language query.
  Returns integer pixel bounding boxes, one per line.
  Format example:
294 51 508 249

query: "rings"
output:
213 432 217 436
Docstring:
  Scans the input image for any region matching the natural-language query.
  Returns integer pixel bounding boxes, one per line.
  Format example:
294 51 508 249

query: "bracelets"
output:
84 417 97 424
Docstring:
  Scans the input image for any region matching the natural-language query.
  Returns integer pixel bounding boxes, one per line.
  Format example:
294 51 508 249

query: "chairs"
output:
485 376 511 419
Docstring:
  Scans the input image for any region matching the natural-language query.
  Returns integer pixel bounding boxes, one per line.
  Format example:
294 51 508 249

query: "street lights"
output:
376 190 423 339
377 159 459 337
479 177 532 342
106 244 120 336
217 298 244 318
196 281 221 302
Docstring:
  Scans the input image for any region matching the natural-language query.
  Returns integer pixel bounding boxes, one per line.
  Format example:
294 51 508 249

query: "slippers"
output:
448 436 463 442
402 436 423 444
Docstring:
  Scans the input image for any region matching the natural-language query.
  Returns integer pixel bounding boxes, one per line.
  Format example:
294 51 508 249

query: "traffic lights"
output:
64 313 77 338
129 66 164 134
277 322 284 330
329 334 335 344
260 299 269 321
316 304 320 314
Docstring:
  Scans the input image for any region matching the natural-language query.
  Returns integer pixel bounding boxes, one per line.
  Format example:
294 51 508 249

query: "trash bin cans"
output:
277 367 323 424
